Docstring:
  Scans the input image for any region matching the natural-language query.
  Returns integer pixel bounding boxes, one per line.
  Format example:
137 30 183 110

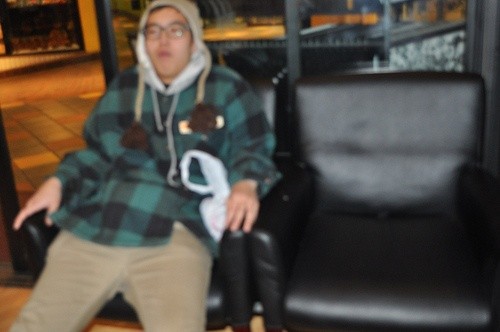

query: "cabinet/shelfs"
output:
95 0 469 88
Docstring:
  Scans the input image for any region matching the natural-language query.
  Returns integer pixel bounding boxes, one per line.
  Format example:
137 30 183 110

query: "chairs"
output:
250 71 500 332
22 76 287 330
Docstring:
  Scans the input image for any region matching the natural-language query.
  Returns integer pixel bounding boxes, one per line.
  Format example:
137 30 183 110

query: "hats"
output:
121 0 217 146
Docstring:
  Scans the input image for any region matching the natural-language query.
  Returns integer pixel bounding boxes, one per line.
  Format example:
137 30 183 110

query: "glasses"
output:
144 22 189 41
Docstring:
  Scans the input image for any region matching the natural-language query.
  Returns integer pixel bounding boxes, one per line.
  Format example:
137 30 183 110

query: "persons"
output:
7 0 283 332
